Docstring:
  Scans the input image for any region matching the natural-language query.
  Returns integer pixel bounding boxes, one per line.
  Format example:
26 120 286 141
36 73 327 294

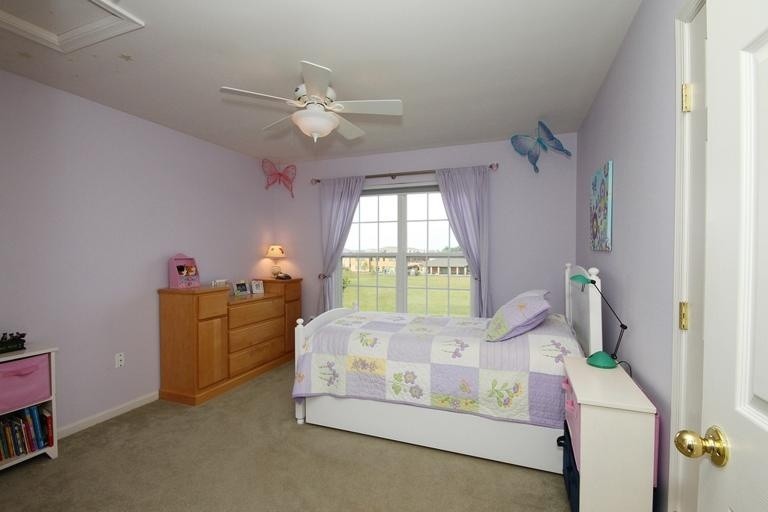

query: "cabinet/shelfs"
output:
1 332 61 469
156 275 305 407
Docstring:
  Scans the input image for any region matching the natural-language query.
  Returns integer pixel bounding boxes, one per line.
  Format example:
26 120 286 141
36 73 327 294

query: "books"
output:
0 404 53 463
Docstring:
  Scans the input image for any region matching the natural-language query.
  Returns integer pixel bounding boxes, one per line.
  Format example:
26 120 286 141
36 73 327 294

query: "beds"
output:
290 261 604 475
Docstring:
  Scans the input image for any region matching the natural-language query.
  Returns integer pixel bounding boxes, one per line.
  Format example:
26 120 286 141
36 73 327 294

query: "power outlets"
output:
114 351 127 370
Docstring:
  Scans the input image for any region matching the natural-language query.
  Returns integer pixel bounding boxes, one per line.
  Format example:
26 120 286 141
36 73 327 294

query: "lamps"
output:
263 244 286 278
569 273 627 372
290 110 343 146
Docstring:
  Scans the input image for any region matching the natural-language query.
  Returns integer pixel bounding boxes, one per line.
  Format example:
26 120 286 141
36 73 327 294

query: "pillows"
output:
486 287 551 342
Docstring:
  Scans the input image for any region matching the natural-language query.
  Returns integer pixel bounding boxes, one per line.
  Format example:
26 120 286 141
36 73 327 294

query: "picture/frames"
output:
251 280 264 294
588 160 614 255
233 279 251 296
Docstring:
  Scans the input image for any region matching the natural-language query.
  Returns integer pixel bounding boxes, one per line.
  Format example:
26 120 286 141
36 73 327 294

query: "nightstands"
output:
559 355 660 512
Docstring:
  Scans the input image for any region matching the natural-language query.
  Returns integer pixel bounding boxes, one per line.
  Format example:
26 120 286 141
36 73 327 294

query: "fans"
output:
216 58 408 149
